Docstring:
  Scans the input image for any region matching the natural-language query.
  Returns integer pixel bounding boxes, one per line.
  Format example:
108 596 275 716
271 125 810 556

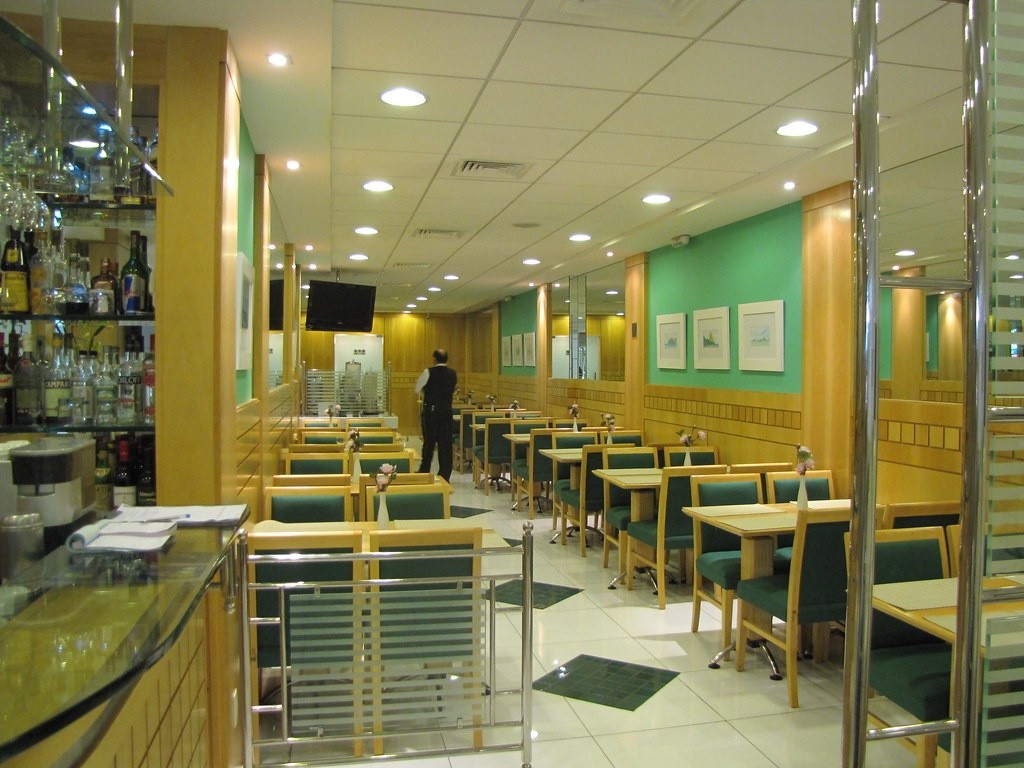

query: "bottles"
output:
89 124 157 205
74 155 91 197
52 147 84 202
121 229 151 316
0 330 156 521
0 225 30 316
23 233 120 314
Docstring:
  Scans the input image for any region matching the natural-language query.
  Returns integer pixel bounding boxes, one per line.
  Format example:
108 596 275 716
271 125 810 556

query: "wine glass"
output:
0 287 18 314
0 136 79 232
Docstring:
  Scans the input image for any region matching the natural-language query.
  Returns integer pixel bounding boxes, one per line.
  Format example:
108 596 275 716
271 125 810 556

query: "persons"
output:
414 349 457 485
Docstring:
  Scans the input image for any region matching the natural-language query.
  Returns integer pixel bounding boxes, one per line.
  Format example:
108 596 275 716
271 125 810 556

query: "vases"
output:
797 473 808 509
352 452 363 483
513 410 518 419
491 403 495 412
376 491 390 530
329 417 333 427
573 417 579 432
684 446 692 466
607 432 613 444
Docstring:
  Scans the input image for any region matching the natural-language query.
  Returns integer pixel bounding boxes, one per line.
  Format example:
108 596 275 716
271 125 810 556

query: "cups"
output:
38 286 66 313
58 397 82 427
89 289 113 313
62 282 88 313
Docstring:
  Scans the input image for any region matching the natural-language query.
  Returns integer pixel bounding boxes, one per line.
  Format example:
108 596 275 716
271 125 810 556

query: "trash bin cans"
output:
1 511 45 578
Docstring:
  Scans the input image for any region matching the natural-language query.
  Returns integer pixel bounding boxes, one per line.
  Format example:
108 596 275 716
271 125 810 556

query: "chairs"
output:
451 398 976 767
252 416 489 767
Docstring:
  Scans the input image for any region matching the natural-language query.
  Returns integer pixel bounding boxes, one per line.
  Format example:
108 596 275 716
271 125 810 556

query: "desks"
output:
503 434 533 511
537 448 587 546
591 469 662 585
681 502 848 661
871 572 1024 767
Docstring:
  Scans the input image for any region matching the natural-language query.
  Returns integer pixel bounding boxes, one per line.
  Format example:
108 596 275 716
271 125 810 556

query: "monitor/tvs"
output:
305 280 377 332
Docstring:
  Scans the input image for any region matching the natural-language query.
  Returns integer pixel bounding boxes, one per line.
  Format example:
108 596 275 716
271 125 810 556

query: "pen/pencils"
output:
144 514 191 520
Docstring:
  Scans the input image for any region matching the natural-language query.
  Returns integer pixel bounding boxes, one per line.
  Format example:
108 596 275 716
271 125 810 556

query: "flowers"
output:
509 400 521 412
675 424 706 447
370 463 398 492
795 443 814 476
567 401 581 419
466 390 475 400
455 389 462 396
344 428 363 454
325 404 341 418
600 412 616 433
486 393 497 405
